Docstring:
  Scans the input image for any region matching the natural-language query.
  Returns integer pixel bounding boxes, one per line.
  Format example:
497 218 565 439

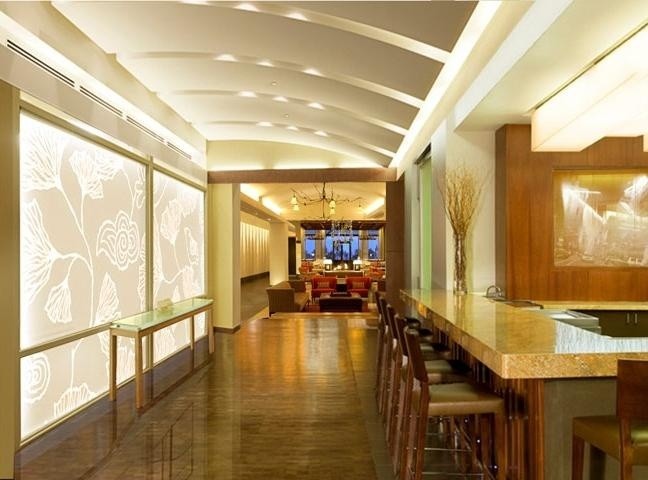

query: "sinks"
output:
488 296 503 299
493 298 504 302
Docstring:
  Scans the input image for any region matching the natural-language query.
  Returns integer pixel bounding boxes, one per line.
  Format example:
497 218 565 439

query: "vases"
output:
453 233 468 296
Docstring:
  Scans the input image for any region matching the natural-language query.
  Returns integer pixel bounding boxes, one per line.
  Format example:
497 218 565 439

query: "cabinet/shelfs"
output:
573 310 648 337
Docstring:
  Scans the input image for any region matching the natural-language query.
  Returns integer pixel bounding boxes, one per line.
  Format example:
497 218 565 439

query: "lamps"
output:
523 19 648 153
287 181 366 247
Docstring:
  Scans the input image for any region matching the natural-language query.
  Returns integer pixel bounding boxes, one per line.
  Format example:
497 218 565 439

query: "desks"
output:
108 297 216 409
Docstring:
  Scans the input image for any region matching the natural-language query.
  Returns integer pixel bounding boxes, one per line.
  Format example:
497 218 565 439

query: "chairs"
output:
572 360 648 480
375 291 506 480
298 261 386 305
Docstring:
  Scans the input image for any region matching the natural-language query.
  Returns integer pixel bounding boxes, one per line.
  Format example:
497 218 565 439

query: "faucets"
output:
486 285 501 297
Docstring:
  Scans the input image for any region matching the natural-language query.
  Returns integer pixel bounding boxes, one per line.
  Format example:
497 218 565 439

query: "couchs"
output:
265 280 310 317
